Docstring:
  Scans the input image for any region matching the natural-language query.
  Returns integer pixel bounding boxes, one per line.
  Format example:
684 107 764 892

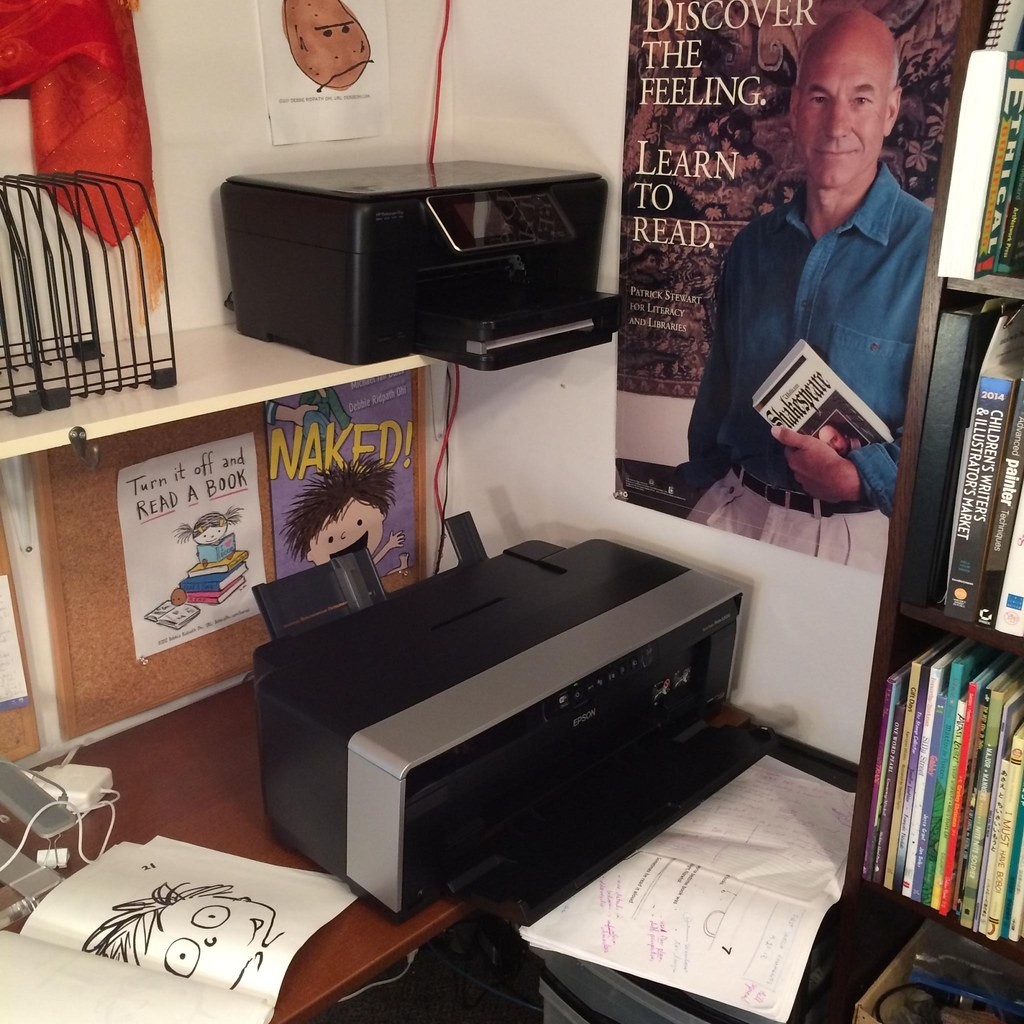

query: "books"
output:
935 42 1024 281
752 338 896 459
858 634 1023 948
993 132 1024 277
903 301 1023 643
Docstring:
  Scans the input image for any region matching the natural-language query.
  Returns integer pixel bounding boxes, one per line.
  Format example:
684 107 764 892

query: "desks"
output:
0 616 754 1024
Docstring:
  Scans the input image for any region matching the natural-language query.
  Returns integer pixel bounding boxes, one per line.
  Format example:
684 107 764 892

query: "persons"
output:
677 6 935 571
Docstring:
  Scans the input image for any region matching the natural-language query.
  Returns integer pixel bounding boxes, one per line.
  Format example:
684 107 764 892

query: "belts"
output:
730 460 882 517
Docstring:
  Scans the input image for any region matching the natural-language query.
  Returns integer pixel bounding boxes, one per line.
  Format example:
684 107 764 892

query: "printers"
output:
219 159 622 370
253 509 780 929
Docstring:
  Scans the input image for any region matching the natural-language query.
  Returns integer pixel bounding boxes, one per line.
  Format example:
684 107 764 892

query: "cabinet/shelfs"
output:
829 0 1024 1024
